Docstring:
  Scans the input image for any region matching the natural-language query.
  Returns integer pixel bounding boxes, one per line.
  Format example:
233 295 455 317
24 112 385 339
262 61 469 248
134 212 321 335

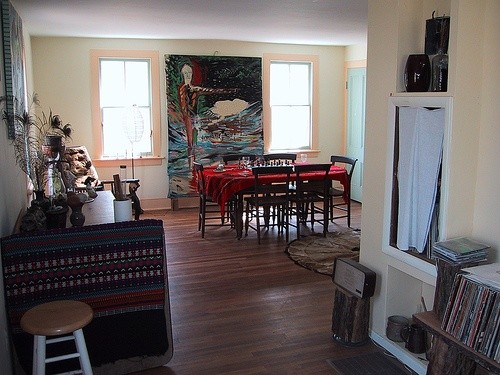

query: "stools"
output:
20 300 93 375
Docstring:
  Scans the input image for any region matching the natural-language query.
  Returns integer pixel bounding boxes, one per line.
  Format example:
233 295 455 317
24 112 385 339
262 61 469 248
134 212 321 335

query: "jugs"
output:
402 320 428 353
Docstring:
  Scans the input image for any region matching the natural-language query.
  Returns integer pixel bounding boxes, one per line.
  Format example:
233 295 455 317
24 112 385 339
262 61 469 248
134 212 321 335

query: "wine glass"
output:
242 156 251 173
301 154 307 165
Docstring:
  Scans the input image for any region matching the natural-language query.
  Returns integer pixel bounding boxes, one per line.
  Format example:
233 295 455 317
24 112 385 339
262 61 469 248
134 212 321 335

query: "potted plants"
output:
1 92 75 214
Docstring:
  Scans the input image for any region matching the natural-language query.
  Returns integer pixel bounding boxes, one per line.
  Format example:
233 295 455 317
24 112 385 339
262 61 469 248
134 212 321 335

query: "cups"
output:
114 198 132 222
387 315 410 342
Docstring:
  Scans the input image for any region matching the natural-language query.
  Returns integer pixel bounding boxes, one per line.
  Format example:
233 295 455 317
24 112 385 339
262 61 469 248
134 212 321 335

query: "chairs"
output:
192 153 358 244
53 145 144 220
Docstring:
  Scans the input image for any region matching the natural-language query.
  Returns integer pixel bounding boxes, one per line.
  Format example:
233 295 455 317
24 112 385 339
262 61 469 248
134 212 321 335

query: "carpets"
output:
325 351 417 375
286 227 361 276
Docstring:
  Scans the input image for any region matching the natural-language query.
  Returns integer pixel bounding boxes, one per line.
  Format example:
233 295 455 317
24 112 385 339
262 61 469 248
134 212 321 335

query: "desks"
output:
412 309 500 375
12 190 117 235
194 162 350 240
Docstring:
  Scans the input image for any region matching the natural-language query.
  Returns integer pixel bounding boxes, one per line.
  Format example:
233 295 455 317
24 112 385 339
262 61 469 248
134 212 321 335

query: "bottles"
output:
431 49 448 92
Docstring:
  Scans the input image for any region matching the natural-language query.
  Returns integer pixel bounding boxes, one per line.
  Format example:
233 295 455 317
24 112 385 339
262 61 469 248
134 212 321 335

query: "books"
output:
441 272 500 365
430 236 490 318
